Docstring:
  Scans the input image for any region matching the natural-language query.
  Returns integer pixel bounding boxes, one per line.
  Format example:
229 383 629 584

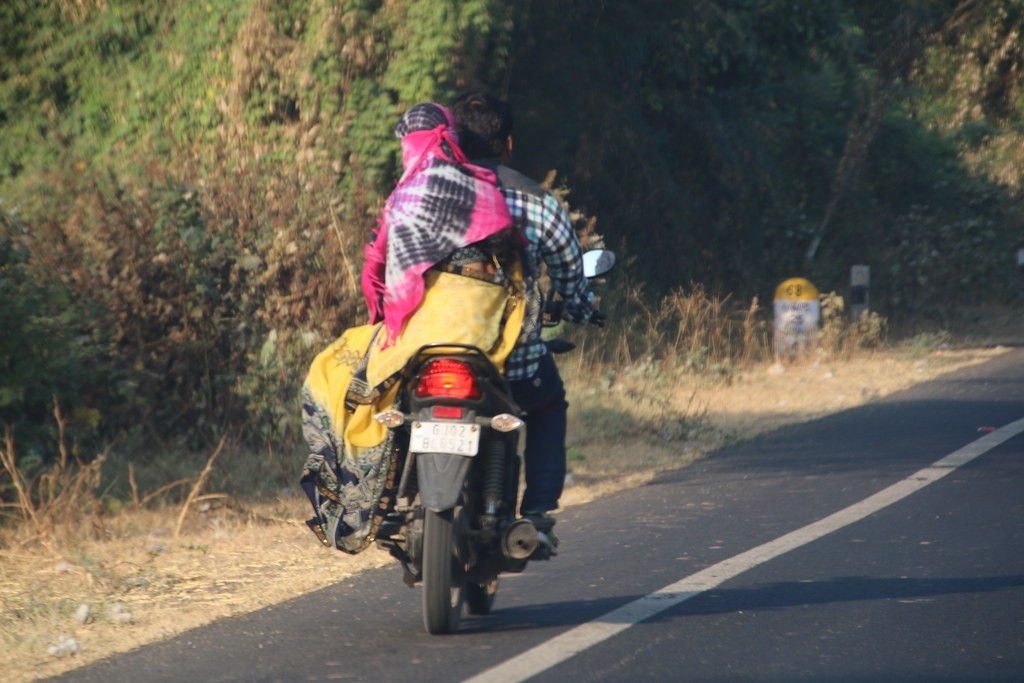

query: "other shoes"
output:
377 510 400 538
520 513 560 556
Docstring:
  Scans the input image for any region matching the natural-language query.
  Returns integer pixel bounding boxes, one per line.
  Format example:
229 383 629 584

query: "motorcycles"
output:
371 247 619 638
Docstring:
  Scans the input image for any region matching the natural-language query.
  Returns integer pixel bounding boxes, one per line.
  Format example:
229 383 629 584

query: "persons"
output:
300 96 596 555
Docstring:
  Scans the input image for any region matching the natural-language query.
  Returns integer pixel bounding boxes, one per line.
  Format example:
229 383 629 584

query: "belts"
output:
431 261 515 288
